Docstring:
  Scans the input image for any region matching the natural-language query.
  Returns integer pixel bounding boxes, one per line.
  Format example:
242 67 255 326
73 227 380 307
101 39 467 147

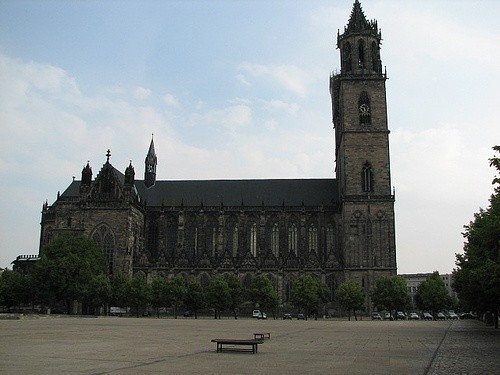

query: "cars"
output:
385 313 394 321
408 313 419 320
258 312 267 320
393 312 406 320
372 313 381 320
0 305 70 314
297 314 305 320
421 312 433 320
142 311 152 317
434 312 445 320
446 312 458 320
184 311 193 317
460 313 476 319
283 314 292 320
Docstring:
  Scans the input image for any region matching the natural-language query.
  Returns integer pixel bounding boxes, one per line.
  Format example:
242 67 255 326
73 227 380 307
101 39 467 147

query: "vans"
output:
253 310 261 318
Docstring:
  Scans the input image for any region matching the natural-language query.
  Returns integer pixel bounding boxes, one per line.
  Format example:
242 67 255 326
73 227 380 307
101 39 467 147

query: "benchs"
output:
254 332 271 341
211 339 264 355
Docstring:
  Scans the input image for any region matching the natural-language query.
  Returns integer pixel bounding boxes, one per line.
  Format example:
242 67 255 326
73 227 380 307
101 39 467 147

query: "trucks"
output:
110 306 131 317
